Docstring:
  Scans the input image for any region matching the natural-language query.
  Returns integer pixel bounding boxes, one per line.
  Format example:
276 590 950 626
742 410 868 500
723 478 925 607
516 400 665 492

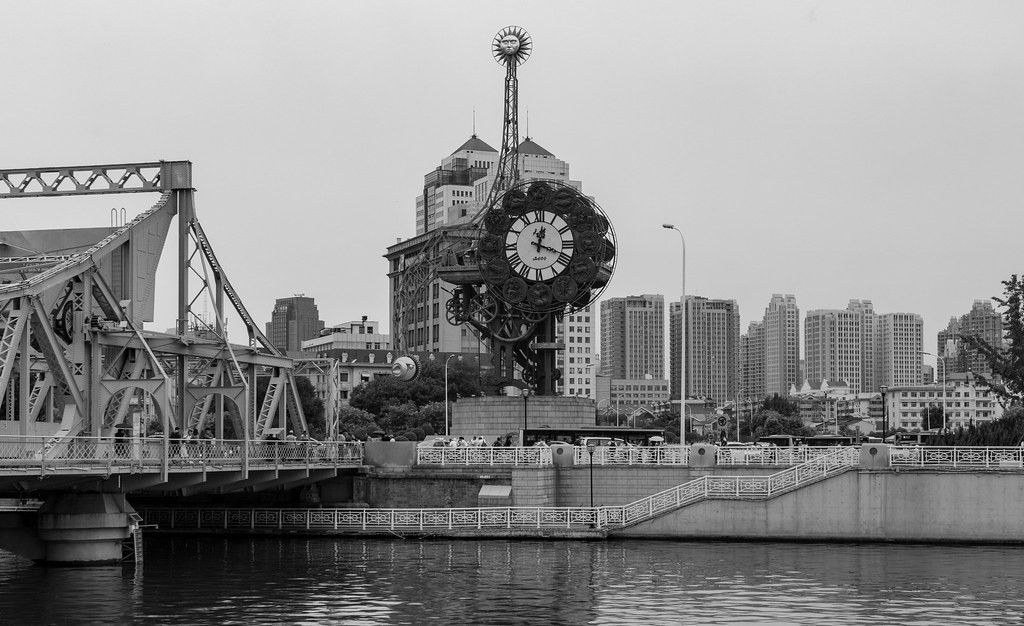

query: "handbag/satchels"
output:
481 440 486 446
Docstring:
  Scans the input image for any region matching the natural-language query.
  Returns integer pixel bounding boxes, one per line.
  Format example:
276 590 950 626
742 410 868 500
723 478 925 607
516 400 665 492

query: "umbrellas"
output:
114 422 132 434
649 435 664 446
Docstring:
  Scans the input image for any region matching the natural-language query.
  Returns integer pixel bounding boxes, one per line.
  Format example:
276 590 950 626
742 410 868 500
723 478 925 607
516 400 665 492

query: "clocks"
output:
505 209 574 284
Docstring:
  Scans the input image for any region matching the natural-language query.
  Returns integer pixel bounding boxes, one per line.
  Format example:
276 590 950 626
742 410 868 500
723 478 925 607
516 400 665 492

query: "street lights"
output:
965 384 976 428
737 388 746 442
617 395 622 426
663 223 685 463
523 389 529 463
919 351 946 429
598 398 609 409
587 444 596 529
855 426 860 443
686 404 692 433
928 398 937 430
445 354 455 436
626 406 635 428
879 385 888 443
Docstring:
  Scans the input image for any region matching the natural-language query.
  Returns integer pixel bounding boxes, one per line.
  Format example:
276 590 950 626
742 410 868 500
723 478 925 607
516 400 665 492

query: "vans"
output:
575 437 637 453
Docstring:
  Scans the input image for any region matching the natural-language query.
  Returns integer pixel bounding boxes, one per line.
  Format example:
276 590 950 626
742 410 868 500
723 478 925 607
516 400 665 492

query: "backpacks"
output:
610 441 616 450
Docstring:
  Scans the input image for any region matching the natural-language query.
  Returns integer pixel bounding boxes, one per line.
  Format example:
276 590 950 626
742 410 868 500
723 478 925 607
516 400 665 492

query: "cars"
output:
533 441 571 458
417 438 450 459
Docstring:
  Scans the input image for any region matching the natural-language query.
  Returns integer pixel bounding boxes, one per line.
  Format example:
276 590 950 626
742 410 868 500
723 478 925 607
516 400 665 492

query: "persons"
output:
265 434 278 445
114 429 132 455
720 427 728 448
337 430 372 460
381 431 396 442
607 437 617 463
837 441 842 448
286 430 296 445
449 436 487 450
323 432 333 462
202 428 213 443
768 441 779 463
182 429 199 444
301 430 308 441
169 427 182 444
492 435 514 448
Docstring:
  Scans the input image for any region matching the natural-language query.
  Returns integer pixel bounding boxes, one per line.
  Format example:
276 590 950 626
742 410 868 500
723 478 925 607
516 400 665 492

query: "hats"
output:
289 430 293 433
175 427 180 430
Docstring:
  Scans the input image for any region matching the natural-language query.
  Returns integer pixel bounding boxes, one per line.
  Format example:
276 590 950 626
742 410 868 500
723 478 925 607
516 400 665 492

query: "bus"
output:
894 432 955 450
753 435 870 453
518 426 666 463
